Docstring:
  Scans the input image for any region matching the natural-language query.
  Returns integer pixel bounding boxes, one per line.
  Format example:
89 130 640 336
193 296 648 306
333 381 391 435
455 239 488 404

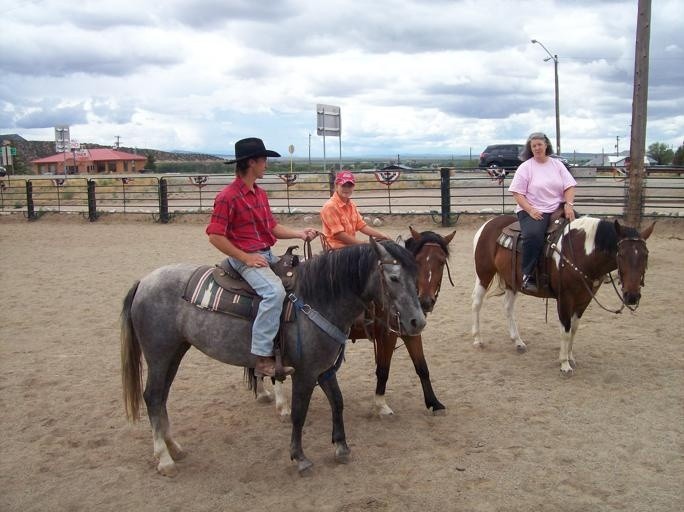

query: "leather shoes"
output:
254 356 296 378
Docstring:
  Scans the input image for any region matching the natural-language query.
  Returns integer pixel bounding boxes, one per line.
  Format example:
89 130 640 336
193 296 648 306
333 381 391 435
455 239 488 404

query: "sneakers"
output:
521 274 538 293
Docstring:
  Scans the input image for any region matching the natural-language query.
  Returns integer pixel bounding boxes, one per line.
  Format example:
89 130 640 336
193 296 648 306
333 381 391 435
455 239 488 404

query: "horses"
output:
121 234 427 479
470 214 657 377
242 225 456 424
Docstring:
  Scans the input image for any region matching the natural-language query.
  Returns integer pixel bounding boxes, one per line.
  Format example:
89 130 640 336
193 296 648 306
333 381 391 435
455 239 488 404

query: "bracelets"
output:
568 202 574 207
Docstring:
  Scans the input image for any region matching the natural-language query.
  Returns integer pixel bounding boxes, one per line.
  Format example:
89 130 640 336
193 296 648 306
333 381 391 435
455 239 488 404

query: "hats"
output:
223 137 281 164
334 170 355 186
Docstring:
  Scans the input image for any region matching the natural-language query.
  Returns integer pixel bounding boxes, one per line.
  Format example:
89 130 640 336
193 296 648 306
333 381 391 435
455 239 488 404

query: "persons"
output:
319 170 390 252
205 137 319 377
507 132 578 291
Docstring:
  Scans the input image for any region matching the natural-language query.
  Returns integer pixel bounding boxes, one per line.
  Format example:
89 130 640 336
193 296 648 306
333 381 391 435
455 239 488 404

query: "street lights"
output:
532 37 561 155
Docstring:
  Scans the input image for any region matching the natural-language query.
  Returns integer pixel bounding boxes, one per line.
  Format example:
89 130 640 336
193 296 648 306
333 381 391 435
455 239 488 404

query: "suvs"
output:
478 144 569 176
